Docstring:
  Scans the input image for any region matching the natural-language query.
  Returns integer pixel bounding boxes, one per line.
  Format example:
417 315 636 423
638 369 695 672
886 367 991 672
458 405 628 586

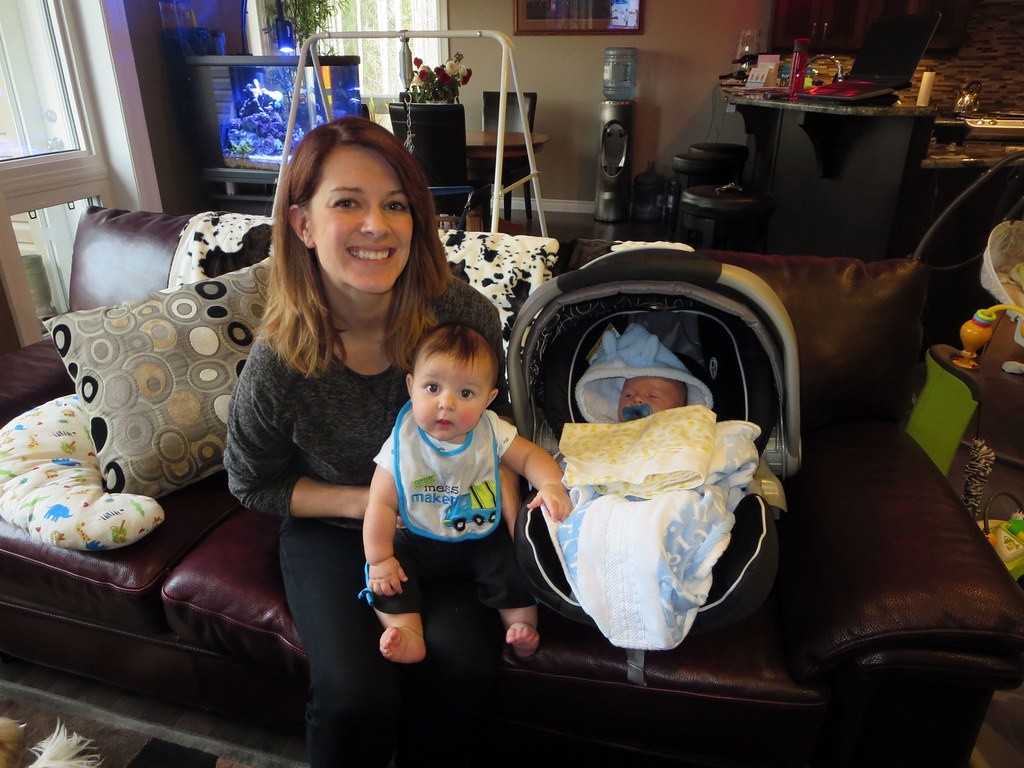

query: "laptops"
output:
792 11 942 102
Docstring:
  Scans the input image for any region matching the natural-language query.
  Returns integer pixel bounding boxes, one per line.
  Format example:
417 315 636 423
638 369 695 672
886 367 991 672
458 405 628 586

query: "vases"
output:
425 100 455 106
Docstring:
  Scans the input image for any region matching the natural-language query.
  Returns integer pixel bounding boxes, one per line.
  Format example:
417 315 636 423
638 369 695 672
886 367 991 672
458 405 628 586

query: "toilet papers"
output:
915 71 937 106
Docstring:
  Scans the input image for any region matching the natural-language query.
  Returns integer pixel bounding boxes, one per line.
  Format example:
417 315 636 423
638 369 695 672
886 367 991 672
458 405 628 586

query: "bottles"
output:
787 38 811 96
630 161 677 221
601 47 638 101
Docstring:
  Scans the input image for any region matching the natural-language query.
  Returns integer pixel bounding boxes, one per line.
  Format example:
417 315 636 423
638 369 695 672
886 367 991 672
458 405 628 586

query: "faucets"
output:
794 53 844 83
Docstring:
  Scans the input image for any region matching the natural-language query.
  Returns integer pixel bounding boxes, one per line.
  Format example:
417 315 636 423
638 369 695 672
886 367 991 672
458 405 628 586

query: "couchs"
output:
0 207 1023 768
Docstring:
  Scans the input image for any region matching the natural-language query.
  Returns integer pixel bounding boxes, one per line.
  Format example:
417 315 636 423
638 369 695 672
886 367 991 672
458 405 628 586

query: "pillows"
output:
40 260 278 499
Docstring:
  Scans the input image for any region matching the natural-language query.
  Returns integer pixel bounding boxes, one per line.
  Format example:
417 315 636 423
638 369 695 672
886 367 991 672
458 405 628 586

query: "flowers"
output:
405 54 471 105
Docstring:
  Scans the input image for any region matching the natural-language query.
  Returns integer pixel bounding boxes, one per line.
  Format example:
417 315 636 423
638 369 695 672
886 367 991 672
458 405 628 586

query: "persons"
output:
549 325 765 650
360 313 571 662
220 116 499 767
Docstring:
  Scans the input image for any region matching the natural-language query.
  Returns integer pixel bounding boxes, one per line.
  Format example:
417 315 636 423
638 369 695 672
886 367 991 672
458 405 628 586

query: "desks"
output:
717 86 937 262
465 131 551 227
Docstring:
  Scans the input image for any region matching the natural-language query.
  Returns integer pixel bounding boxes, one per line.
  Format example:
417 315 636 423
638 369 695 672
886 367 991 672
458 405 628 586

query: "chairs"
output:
389 103 491 231
482 92 538 225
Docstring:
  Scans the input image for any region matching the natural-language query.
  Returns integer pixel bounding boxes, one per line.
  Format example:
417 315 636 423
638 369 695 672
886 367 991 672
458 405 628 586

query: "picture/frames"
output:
513 0 642 35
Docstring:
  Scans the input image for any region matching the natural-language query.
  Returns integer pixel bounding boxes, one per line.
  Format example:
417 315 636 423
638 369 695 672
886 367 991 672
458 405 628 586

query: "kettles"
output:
951 80 985 118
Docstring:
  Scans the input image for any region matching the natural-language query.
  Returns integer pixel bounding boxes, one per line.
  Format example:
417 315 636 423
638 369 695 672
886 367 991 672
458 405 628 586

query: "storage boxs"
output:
186 53 361 175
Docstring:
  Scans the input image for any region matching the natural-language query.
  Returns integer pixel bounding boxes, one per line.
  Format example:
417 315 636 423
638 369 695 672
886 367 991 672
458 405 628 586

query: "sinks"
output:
964 119 1024 139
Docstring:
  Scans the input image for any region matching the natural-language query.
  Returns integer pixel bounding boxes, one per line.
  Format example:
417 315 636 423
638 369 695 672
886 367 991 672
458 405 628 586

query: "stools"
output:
676 142 754 247
